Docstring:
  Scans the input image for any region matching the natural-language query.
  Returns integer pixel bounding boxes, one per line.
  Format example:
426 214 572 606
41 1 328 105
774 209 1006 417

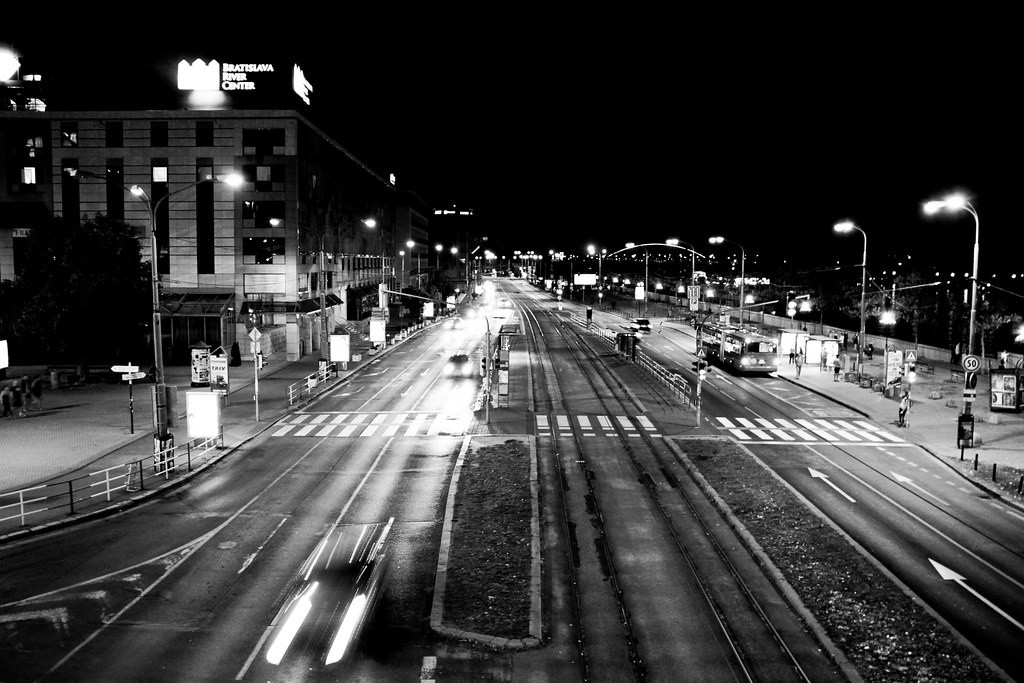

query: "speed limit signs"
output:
962 355 980 372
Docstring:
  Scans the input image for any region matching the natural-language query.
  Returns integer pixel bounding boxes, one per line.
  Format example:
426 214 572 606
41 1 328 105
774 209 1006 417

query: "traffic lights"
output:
899 364 906 382
787 291 796 317
909 365 916 383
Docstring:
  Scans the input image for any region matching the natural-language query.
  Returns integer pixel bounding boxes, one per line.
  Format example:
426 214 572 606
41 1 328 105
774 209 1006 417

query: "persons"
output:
828 330 848 351
853 332 859 350
833 355 841 382
0 374 44 419
898 391 908 428
868 342 874 359
231 342 241 366
789 346 803 379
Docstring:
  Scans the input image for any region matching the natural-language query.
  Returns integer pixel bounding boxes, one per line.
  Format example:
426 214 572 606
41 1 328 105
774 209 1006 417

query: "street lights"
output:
924 194 979 446
709 236 745 328
407 240 420 290
626 243 648 317
743 295 754 331
879 311 897 388
667 239 695 317
269 218 376 382
800 301 812 330
678 286 685 308
435 245 443 271
706 290 714 316
70 170 244 474
587 245 602 307
834 221 866 378
399 251 405 289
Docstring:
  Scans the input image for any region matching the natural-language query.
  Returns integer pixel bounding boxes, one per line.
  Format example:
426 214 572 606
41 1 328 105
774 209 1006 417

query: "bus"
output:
694 322 778 372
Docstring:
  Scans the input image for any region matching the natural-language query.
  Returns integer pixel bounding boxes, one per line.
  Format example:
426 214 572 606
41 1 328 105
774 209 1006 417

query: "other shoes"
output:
795 377 799 380
834 380 839 382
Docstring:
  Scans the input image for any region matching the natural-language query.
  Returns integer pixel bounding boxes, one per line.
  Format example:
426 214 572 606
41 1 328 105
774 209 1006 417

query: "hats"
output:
899 392 905 397
